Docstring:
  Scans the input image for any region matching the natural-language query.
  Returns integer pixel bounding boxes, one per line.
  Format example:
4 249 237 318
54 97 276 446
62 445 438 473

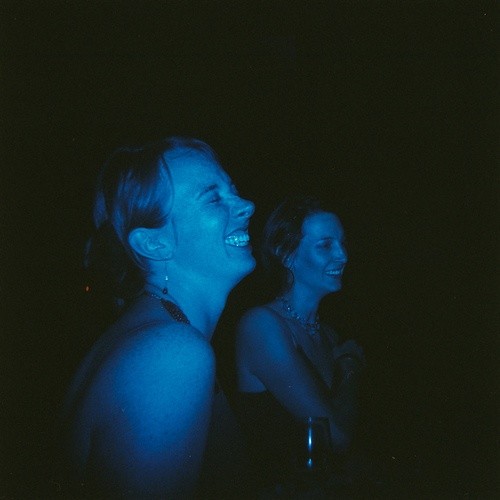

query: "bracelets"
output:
330 352 357 368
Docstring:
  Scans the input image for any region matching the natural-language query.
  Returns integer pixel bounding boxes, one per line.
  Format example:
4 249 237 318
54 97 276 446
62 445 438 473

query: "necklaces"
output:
142 289 192 326
276 293 322 340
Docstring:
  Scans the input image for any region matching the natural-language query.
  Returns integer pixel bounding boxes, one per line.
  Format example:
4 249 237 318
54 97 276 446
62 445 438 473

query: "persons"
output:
38 135 260 500
228 196 368 500
